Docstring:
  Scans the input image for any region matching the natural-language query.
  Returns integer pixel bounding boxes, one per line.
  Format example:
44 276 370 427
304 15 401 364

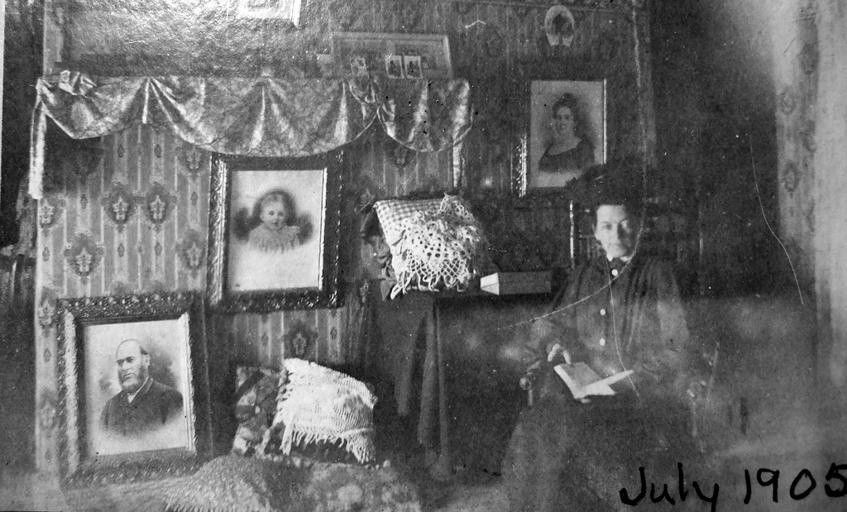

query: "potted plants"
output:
329 29 455 81
204 148 341 316
52 288 210 489
508 66 615 209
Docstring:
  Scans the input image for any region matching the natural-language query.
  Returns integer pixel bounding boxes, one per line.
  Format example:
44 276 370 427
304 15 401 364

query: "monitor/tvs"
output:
551 360 641 400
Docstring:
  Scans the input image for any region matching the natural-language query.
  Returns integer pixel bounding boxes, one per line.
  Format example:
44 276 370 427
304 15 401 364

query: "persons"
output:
249 192 302 253
502 194 701 508
531 91 596 178
99 338 184 434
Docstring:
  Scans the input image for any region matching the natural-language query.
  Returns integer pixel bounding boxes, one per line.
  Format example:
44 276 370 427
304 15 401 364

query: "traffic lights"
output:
372 190 492 298
258 355 379 469
226 359 278 455
